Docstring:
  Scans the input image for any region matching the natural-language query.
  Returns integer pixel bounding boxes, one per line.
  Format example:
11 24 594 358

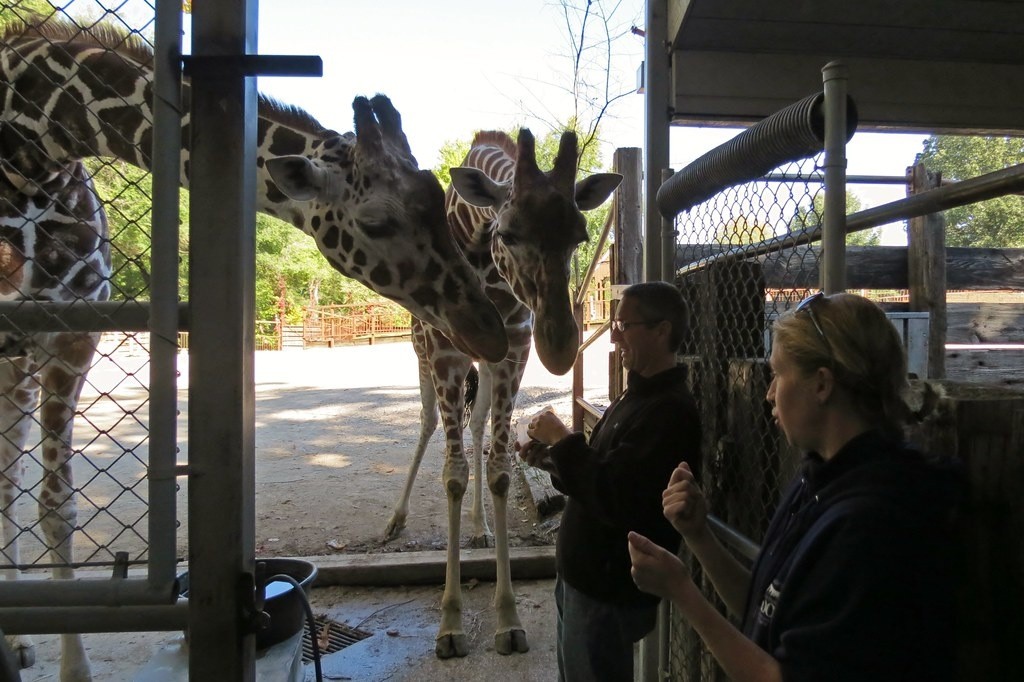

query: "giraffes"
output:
375 129 620 666
0 14 509 682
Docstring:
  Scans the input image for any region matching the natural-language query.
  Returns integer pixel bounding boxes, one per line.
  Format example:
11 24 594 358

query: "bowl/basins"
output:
177 558 319 651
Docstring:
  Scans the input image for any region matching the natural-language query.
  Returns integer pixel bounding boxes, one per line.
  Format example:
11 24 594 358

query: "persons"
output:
628 292 958 682
515 281 703 682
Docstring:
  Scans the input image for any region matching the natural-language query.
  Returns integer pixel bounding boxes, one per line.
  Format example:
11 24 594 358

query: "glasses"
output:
610 320 659 333
794 291 830 350
